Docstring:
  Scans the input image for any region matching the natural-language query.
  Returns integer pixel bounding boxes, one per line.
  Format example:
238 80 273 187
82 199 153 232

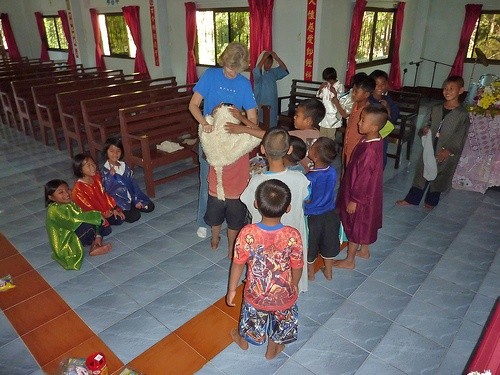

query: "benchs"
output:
0 56 271 198
279 80 423 170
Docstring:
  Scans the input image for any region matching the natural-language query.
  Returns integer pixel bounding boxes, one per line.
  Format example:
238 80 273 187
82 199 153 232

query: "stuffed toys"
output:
192 102 262 202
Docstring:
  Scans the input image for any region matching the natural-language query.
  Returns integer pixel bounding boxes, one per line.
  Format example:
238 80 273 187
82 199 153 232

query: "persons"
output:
331 102 388 269
396 75 470 212
315 66 395 182
239 98 345 281
44 179 112 256
226 179 303 359
71 152 126 235
100 136 155 222
251 50 289 155
189 41 259 238
